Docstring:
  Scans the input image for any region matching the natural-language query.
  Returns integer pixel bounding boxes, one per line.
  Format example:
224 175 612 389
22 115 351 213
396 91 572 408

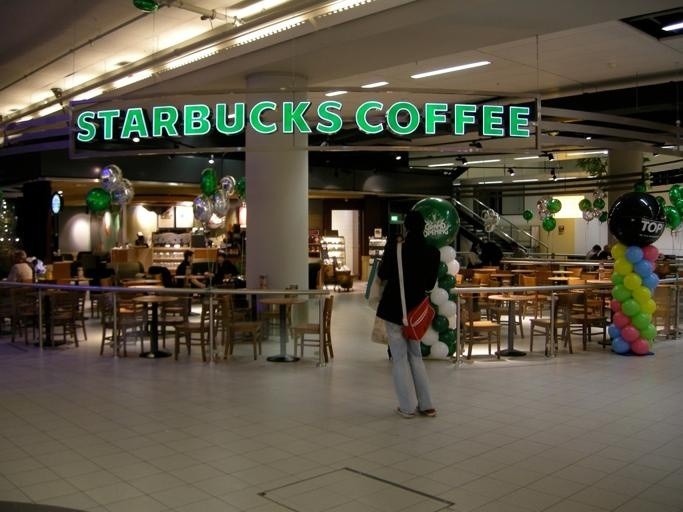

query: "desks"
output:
119 277 161 284
488 294 532 356
548 277 579 281
260 298 308 363
133 295 178 358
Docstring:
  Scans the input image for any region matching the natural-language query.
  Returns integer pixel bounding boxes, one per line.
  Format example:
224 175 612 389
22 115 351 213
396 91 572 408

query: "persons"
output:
376 210 441 419
176 250 205 288
599 245 612 260
585 244 601 261
509 242 526 258
469 242 480 256
6 251 33 284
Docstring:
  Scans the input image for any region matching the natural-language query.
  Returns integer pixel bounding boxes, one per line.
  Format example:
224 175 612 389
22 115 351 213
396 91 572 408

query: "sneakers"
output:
395 406 415 418
416 406 435 417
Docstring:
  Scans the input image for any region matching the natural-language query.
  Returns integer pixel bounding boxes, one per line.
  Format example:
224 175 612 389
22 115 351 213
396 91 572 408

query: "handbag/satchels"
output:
397 242 435 340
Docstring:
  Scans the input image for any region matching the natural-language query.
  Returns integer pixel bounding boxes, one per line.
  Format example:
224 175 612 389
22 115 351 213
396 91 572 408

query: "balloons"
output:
542 217 556 232
577 188 608 224
631 285 648 304
625 245 643 263
632 339 649 355
480 208 500 233
611 299 621 312
621 299 641 317
411 197 460 250
109 178 135 206
212 189 231 218
611 242 628 260
234 176 246 202
643 245 659 263
613 258 633 276
606 192 667 249
99 164 123 192
536 196 553 214
639 299 655 314
623 273 641 291
612 285 634 300
85 187 112 212
632 312 651 331
641 324 657 340
219 174 236 197
668 184 683 206
610 272 625 284
193 192 214 230
632 180 646 193
633 260 651 278
547 198 561 215
662 206 681 231
522 209 534 222
199 168 218 197
421 246 462 361
621 326 640 343
611 337 631 355
642 273 659 288
608 324 620 340
674 197 683 217
613 313 631 327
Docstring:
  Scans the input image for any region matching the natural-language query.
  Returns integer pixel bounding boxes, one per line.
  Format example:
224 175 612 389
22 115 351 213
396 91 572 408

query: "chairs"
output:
100 277 130 304
565 292 608 352
11 290 40 346
50 288 87 348
293 295 334 362
460 295 501 360
223 293 264 361
530 292 578 357
490 291 526 338
522 275 547 319
98 292 144 358
175 302 222 362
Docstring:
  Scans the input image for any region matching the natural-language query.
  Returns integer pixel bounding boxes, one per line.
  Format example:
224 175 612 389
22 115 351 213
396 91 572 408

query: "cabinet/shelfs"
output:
320 234 345 268
152 248 193 271
